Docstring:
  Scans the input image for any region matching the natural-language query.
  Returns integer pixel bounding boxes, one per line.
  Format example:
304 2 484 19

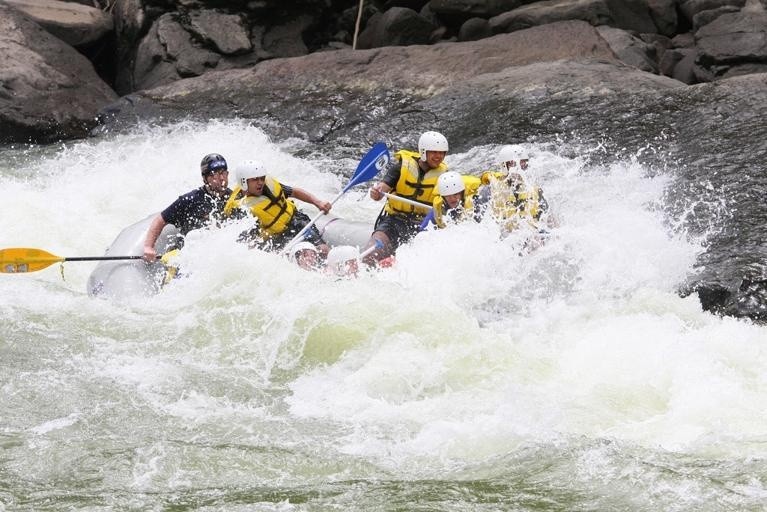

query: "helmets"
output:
438 171 465 205
418 131 448 162
496 145 529 176
236 160 267 191
201 153 227 183
289 242 319 264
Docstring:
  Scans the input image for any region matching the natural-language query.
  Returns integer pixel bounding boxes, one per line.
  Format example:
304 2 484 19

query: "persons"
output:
290 243 316 269
222 162 332 259
359 131 449 266
474 146 560 253
418 171 490 232
144 153 236 260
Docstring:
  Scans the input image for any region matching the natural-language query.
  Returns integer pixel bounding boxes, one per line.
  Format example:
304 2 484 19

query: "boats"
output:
85 206 574 363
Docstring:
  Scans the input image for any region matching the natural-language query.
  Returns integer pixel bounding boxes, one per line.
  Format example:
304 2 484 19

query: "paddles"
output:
284 141 389 250
0 249 164 274
